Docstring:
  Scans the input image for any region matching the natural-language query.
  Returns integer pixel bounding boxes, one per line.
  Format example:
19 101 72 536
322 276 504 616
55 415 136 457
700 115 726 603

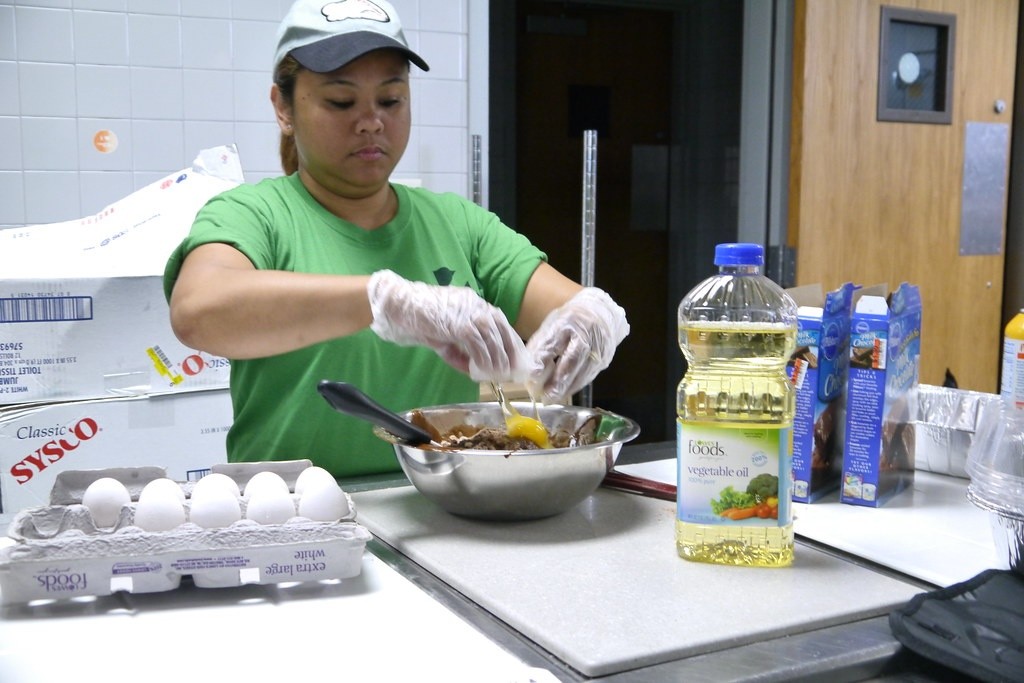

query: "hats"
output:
273 0 429 74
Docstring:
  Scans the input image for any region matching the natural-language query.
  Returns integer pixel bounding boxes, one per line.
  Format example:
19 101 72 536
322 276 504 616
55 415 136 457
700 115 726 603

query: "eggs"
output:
490 381 554 449
134 466 349 533
82 478 132 529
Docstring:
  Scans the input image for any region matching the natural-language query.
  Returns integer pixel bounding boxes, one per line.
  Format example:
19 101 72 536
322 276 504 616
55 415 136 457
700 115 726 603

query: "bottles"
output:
676 244 796 567
1002 308 1024 398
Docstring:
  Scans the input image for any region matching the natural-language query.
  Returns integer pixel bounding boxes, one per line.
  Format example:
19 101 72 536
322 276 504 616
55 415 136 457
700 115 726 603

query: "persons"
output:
163 1 631 480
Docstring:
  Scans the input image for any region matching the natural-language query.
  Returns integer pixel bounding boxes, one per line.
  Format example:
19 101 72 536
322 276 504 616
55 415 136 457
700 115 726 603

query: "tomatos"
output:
755 503 778 519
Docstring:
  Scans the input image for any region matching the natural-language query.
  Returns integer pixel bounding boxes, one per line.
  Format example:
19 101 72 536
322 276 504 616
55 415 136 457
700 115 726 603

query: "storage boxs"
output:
0 387 235 517
783 284 867 503
840 282 923 507
916 384 1001 480
0 143 247 408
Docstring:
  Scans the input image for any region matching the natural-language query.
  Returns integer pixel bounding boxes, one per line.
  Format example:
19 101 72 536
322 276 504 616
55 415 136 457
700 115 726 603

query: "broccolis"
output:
746 473 778 504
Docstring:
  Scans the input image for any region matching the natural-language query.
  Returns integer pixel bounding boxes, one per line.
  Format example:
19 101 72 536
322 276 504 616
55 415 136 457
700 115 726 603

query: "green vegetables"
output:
710 486 755 514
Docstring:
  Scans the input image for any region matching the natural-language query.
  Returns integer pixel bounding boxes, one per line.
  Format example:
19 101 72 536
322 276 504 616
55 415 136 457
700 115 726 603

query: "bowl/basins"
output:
373 401 642 520
964 399 1024 571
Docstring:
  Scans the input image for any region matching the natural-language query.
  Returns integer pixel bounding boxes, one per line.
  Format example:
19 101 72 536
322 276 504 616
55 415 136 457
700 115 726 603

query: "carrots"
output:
721 507 757 519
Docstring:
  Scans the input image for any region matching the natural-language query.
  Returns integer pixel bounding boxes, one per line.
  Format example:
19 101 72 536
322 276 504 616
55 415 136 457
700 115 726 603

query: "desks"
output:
0 449 1012 683
